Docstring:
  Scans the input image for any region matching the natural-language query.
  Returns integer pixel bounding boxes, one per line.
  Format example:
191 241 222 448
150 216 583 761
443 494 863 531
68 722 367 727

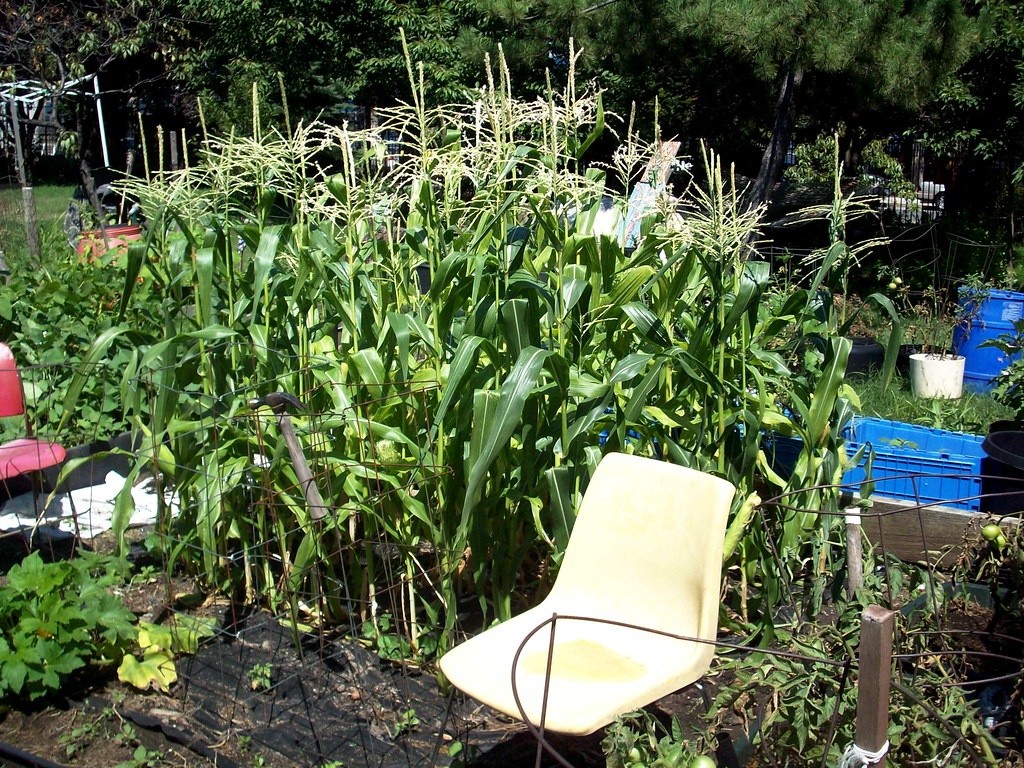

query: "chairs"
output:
0 341 67 505
440 450 739 768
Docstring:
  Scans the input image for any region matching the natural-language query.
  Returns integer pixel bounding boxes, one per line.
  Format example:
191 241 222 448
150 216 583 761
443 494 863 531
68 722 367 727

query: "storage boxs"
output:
600 280 1024 511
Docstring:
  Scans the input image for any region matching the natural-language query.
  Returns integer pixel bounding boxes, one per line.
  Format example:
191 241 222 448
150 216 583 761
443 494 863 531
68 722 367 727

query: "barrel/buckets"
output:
956 286 1024 396
79 225 142 265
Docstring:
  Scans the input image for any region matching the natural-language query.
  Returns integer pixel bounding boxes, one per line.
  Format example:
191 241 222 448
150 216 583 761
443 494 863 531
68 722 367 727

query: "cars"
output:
916 181 950 210
